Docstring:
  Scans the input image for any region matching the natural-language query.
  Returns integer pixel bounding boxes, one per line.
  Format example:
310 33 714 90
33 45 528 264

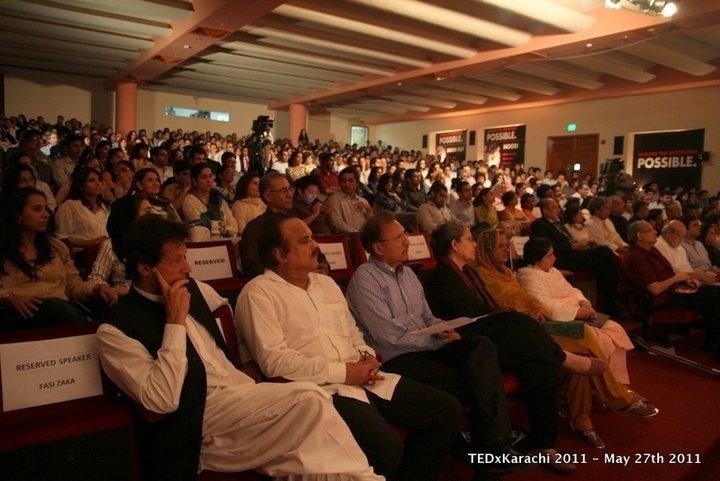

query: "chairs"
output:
576 267 705 348
2 324 142 479
169 229 448 383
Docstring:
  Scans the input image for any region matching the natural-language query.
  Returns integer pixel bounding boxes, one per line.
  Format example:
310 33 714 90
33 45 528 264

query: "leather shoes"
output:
566 353 608 376
527 446 577 473
619 394 660 418
578 429 606 449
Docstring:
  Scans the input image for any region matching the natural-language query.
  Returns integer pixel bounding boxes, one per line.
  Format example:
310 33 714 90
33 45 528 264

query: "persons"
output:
232 212 465 481
346 210 528 479
467 227 660 453
515 236 648 414
94 210 384 480
1 110 720 370
422 219 613 475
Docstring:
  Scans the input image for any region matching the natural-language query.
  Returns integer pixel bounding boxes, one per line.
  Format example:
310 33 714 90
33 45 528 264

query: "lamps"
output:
604 0 678 18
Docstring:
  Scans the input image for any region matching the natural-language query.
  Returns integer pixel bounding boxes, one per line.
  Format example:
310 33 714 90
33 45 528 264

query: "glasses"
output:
269 186 294 193
375 230 410 241
359 348 374 360
